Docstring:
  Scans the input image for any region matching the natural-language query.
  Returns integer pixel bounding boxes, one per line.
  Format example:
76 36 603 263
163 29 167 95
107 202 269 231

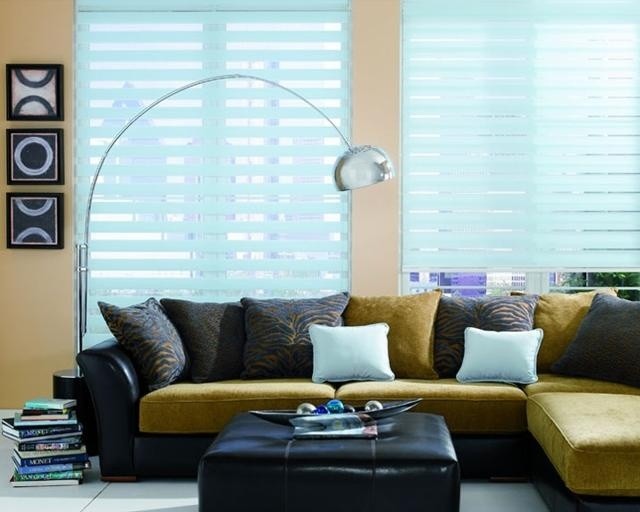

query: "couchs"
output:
76 288 640 512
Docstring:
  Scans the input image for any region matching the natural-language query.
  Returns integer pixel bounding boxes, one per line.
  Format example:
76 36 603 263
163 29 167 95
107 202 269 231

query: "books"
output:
24 399 77 409
2 409 92 487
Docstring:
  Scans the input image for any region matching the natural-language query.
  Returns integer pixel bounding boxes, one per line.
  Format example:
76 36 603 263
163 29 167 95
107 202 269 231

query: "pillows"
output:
456 327 544 383
344 289 440 379
245 296 347 377
98 300 185 385
309 322 395 383
439 295 536 374
519 289 618 374
554 295 640 380
165 301 243 381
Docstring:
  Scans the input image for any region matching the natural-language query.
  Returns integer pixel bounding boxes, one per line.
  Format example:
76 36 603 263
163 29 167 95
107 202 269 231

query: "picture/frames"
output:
5 64 64 120
6 192 64 249
5 128 64 185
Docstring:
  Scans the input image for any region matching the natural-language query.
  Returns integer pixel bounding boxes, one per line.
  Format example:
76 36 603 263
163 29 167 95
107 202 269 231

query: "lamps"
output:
53 74 394 453
